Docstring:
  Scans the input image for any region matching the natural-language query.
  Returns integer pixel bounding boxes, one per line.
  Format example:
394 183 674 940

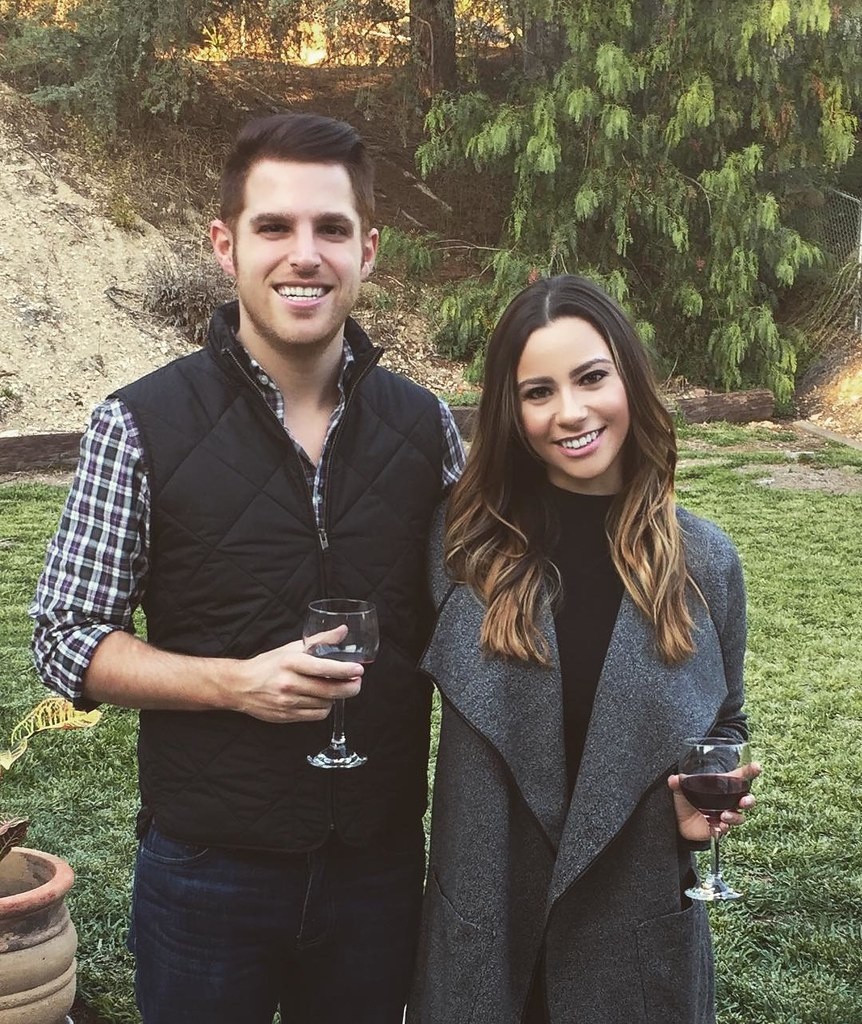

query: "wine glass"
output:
679 737 752 902
302 597 378 771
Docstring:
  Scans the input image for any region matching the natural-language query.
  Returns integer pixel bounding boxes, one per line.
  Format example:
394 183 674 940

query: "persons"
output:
404 272 762 1024
31 117 467 1024
